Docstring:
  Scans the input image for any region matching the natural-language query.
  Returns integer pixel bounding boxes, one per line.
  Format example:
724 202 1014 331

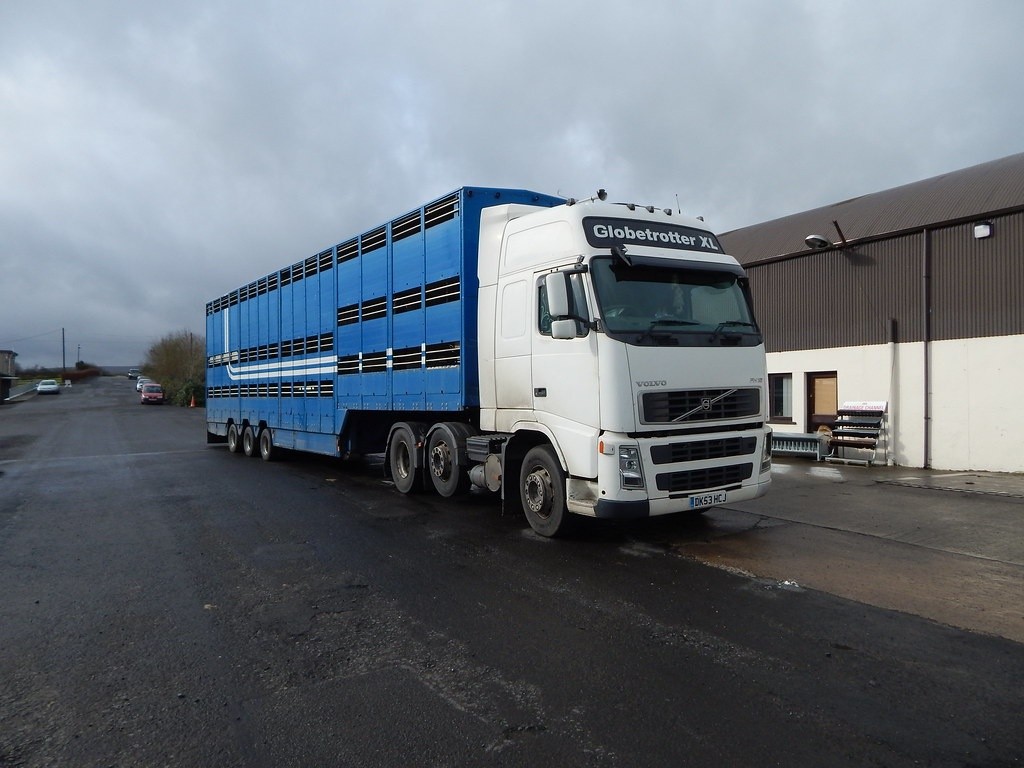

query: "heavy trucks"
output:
206 185 774 538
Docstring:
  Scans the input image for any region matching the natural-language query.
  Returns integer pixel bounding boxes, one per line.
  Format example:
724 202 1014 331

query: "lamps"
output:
805 234 849 252
973 220 992 240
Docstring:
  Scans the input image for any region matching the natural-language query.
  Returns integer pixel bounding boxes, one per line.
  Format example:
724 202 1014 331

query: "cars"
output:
136 376 153 392
127 369 143 379
36 379 61 395
141 384 166 405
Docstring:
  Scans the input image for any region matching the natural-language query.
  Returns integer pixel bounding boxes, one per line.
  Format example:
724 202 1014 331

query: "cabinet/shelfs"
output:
826 399 892 470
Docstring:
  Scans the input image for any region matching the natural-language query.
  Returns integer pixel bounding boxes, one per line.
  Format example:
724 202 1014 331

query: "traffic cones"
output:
191 395 196 407
